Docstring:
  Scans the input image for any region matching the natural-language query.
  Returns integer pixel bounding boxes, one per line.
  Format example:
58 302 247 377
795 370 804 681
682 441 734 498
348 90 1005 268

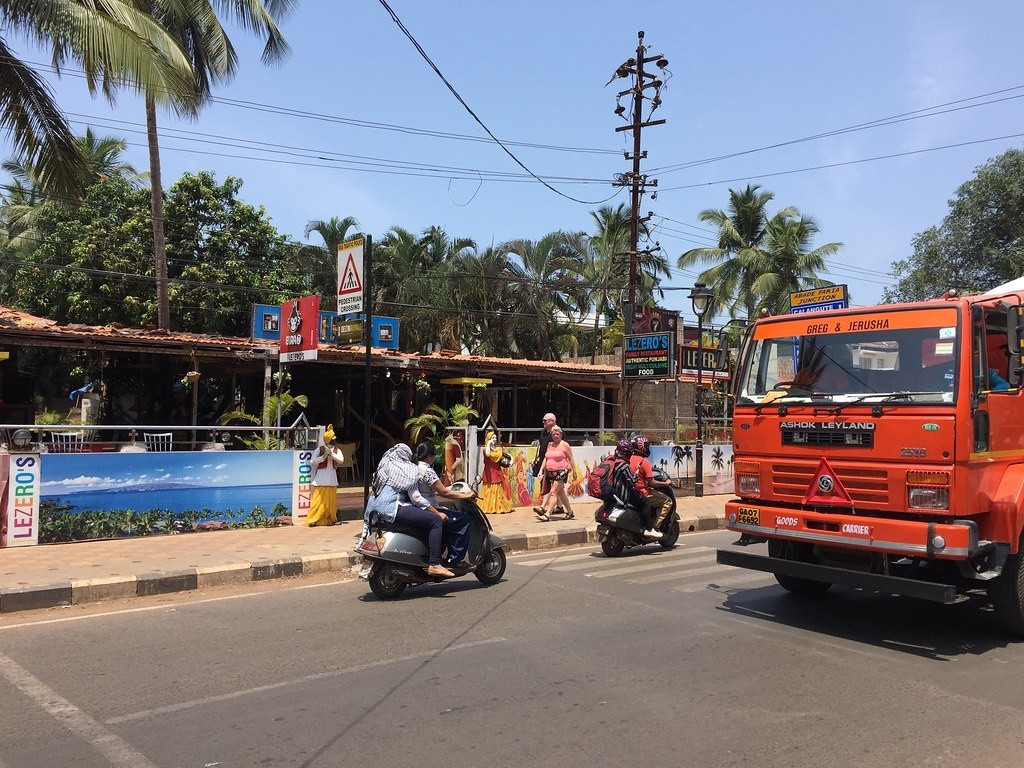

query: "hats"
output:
323 430 336 444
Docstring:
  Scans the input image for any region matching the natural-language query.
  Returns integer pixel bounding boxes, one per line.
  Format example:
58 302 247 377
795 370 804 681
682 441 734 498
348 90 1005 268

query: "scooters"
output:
595 469 680 557
354 475 507 599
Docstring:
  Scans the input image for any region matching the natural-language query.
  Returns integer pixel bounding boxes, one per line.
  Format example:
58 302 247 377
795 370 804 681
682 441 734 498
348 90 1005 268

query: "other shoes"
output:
428 565 455 577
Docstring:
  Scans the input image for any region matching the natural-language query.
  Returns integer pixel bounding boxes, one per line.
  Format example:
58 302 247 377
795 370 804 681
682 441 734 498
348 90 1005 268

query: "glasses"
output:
542 420 551 422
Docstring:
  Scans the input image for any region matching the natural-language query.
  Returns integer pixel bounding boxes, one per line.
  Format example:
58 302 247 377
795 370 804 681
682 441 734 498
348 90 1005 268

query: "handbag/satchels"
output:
532 459 541 477
540 467 550 495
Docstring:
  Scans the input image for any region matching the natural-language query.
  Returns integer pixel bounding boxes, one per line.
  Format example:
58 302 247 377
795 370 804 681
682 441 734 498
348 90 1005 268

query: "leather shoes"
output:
444 555 476 569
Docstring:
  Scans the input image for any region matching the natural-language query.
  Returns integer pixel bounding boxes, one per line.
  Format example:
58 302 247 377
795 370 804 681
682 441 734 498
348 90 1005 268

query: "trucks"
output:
711 289 1023 638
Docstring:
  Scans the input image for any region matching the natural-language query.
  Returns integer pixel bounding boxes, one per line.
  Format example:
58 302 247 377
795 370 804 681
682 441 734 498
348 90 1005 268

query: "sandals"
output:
551 506 564 514
533 507 544 515
537 515 550 521
564 512 575 520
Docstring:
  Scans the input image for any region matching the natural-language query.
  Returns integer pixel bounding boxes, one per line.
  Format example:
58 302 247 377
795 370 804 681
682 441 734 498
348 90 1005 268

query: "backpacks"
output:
588 456 626 499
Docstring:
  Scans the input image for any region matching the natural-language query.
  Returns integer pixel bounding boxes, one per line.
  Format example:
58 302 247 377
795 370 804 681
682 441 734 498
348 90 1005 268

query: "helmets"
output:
616 439 633 461
630 435 650 457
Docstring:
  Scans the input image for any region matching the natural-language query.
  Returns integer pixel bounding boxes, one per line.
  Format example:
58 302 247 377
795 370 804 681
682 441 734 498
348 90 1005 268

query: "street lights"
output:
687 282 714 497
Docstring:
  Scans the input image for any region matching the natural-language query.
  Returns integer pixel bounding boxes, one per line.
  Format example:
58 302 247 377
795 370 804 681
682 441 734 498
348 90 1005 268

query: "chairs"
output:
143 433 172 452
334 440 360 482
51 431 83 453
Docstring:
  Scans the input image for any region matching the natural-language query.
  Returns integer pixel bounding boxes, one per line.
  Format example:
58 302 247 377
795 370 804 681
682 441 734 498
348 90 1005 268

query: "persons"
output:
533 414 578 521
788 347 848 393
612 440 663 538
630 435 673 535
973 340 1010 391
306 426 344 526
477 432 514 513
364 443 455 577
414 442 476 569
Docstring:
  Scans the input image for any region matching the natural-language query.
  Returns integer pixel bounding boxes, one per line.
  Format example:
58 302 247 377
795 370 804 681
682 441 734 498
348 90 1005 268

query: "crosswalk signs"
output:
339 254 362 295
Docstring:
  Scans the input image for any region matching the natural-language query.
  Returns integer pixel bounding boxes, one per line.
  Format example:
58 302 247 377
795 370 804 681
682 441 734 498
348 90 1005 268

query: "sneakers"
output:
644 529 659 538
650 528 663 537
